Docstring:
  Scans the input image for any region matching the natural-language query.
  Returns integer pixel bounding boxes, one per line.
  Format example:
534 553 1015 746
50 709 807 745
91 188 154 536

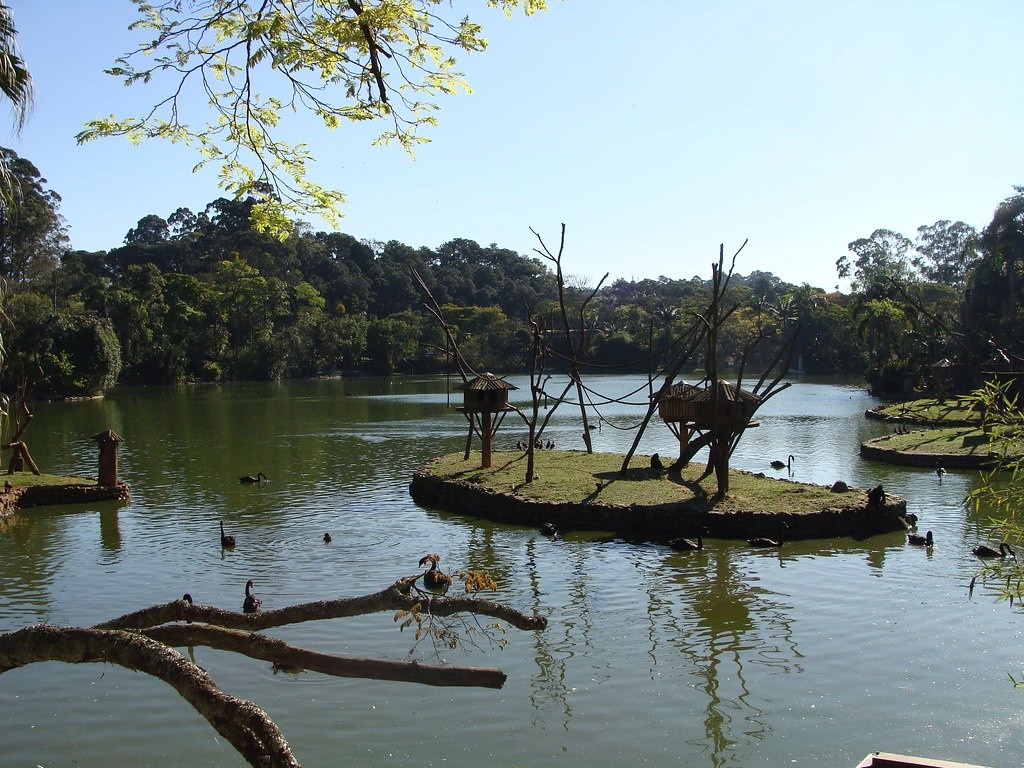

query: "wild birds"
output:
542 521 558 537
650 453 664 468
220 522 235 548
243 581 261 613
418 556 448 587
587 418 603 428
670 526 710 551
973 542 1015 558
908 531 933 545
596 483 605 492
770 455 794 467
323 533 331 542
935 457 946 473
515 438 555 449
239 472 267 483
747 521 789 547
